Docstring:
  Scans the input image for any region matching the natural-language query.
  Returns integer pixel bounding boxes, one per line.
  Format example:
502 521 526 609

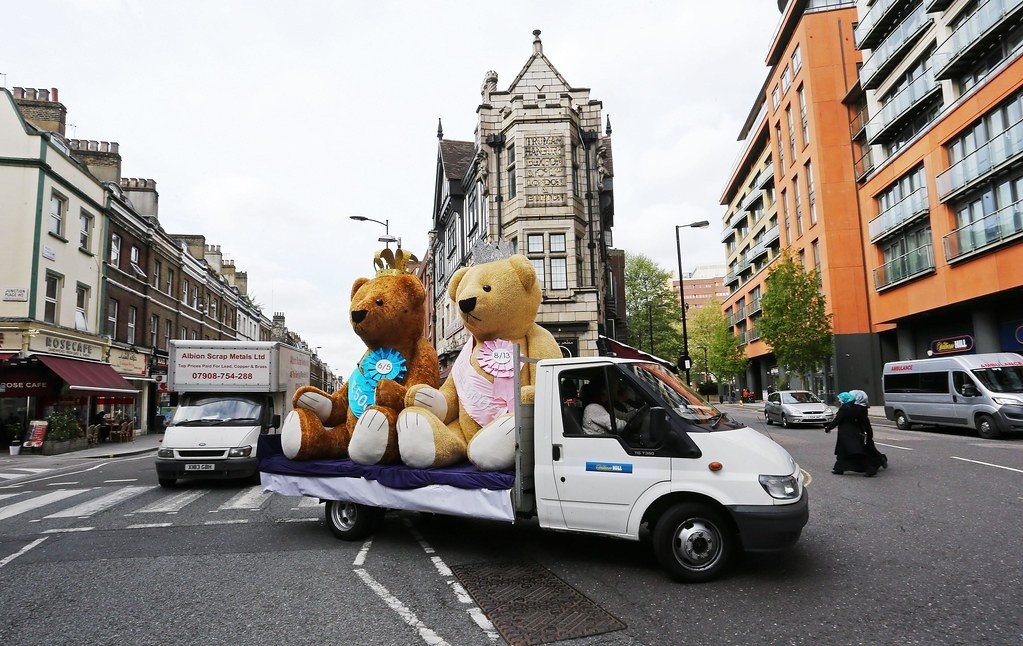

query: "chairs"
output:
559 379 593 436
86 423 101 448
109 420 134 444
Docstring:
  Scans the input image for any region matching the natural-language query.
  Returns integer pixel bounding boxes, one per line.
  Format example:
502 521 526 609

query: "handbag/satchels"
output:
858 431 867 445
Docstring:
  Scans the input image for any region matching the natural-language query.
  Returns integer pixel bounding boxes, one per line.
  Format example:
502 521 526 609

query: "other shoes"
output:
864 471 876 477
881 454 887 468
832 470 843 475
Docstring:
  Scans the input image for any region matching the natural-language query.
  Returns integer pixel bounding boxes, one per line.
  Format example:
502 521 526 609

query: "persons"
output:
91 410 106 441
824 391 879 478
579 381 639 442
847 389 889 471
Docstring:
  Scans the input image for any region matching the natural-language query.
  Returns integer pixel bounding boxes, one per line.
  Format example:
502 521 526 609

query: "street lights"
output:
350 214 389 270
696 345 710 403
675 219 711 390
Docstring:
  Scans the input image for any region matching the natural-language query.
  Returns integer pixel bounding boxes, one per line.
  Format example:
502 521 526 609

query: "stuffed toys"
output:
282 249 440 465
395 254 565 470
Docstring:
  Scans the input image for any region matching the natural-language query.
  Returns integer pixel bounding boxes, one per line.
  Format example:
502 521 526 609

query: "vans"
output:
882 352 1022 439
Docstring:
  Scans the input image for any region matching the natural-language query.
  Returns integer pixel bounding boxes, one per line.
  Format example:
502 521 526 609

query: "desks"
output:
102 424 120 444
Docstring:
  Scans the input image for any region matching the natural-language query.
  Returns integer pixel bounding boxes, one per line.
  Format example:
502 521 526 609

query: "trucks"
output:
259 343 810 583
156 341 335 490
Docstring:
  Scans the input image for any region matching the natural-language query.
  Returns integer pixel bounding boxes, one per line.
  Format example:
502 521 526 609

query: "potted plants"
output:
43 411 89 456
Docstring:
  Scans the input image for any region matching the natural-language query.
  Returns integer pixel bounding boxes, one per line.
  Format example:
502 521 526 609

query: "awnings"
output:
597 335 680 379
31 351 143 396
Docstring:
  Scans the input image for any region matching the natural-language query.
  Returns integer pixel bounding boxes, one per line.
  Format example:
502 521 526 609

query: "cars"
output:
764 390 834 429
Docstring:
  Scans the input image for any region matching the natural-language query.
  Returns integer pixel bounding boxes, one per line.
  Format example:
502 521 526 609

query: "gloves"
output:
824 425 830 433
